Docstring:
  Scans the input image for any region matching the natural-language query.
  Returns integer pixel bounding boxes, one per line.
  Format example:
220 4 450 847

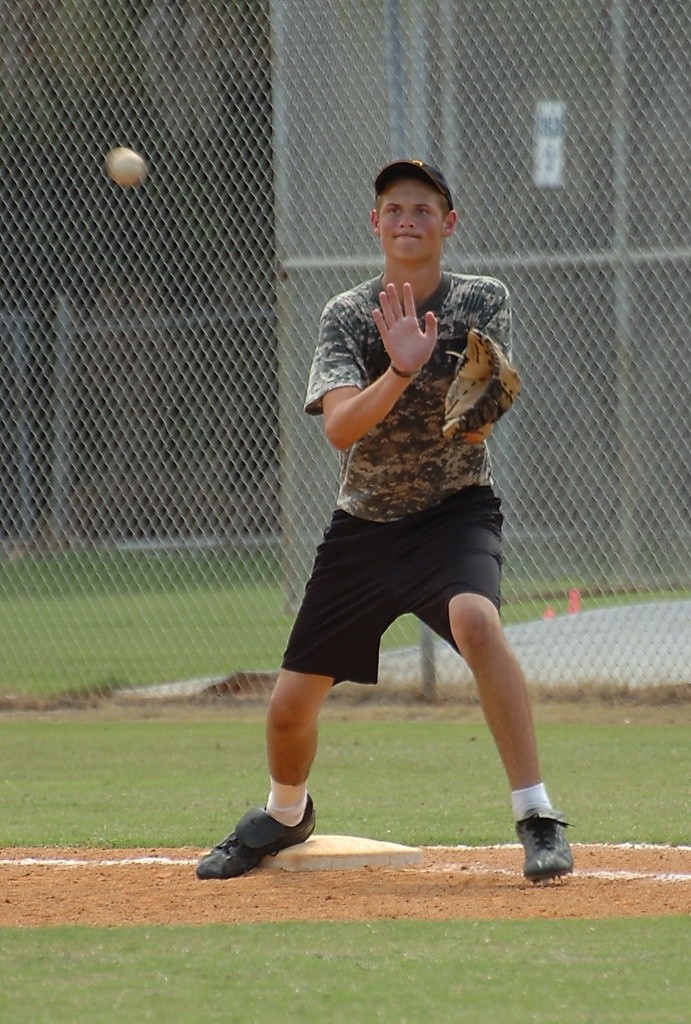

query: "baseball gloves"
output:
441 326 522 444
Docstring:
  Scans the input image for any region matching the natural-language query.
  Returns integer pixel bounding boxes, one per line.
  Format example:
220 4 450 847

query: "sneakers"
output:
195 793 316 880
515 806 576 886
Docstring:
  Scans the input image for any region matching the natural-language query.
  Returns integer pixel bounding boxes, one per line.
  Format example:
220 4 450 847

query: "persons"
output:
196 161 575 883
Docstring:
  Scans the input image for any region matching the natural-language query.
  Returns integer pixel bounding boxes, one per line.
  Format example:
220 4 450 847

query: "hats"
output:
374 159 453 211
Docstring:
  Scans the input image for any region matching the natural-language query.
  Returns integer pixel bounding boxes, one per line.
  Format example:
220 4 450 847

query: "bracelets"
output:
390 363 421 378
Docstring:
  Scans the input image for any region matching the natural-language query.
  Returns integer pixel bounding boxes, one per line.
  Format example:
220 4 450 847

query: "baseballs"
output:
110 153 146 185
